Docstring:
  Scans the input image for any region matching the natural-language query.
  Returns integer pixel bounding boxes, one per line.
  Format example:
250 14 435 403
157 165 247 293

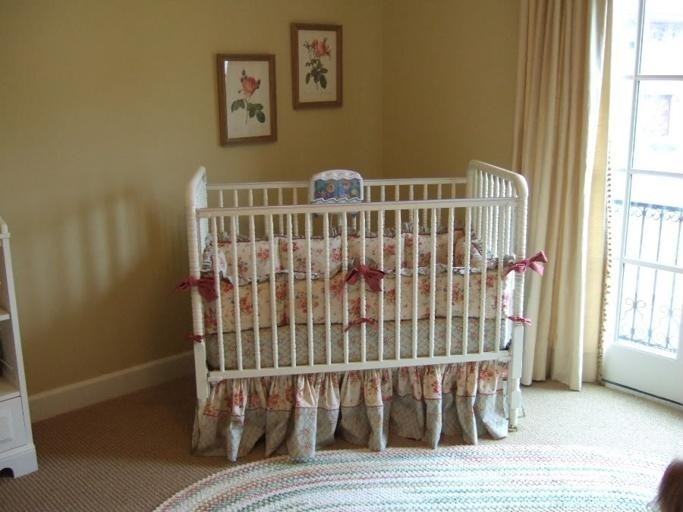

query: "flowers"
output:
301 36 331 94
229 69 267 128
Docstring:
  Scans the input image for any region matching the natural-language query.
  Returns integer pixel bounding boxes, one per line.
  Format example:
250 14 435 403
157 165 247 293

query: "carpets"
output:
151 444 683 512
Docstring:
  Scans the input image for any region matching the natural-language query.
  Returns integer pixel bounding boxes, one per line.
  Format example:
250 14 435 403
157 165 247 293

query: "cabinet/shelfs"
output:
0 216 39 481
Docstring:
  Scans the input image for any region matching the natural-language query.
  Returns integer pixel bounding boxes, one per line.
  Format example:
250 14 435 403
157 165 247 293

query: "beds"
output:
174 157 549 464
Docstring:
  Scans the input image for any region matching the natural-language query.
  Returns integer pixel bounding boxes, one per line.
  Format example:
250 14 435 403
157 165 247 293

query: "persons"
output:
644 458 683 512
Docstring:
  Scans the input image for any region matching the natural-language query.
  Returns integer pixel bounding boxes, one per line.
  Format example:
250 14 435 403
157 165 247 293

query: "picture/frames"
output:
288 20 344 111
216 51 278 146
